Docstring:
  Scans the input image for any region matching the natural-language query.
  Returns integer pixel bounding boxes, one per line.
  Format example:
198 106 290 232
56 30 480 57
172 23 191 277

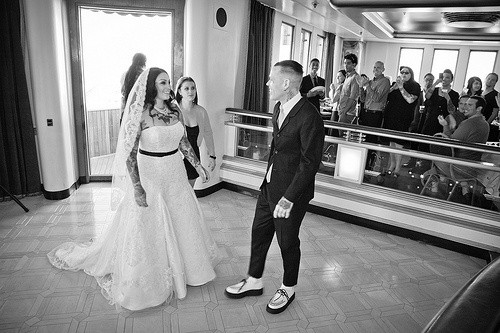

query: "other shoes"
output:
401 163 415 168
266 285 296 314
225 279 264 299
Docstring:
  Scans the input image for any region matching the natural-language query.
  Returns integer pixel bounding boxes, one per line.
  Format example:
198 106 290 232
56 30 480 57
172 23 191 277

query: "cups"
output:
442 89 448 98
419 105 425 113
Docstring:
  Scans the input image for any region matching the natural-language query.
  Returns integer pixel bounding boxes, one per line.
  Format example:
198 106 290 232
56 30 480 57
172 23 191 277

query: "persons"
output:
119 53 148 126
224 61 324 314
47 67 223 316
174 75 216 188
300 59 325 111
328 53 500 205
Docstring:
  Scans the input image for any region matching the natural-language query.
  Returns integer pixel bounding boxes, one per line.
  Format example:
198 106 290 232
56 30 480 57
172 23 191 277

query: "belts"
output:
363 109 381 114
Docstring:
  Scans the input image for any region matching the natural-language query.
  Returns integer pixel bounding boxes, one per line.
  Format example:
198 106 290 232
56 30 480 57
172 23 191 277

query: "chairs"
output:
419 133 476 206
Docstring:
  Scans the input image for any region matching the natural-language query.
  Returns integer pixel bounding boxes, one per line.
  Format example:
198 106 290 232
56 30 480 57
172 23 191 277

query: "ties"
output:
266 106 284 183
313 77 317 87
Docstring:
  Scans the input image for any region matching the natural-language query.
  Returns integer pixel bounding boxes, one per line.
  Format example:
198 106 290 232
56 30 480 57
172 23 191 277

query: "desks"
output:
476 142 500 212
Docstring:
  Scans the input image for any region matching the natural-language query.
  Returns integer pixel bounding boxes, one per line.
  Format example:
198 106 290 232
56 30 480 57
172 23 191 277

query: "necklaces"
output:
155 105 167 114
149 106 171 124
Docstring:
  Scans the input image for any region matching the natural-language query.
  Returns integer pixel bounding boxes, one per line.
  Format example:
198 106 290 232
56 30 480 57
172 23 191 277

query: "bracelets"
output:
210 156 216 159
133 181 141 186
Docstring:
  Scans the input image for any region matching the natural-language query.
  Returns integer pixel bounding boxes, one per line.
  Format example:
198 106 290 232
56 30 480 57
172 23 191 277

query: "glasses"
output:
400 71 409 75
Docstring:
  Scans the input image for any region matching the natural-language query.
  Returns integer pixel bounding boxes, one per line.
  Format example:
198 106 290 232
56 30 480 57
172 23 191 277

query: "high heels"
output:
386 168 395 175
393 172 400 179
